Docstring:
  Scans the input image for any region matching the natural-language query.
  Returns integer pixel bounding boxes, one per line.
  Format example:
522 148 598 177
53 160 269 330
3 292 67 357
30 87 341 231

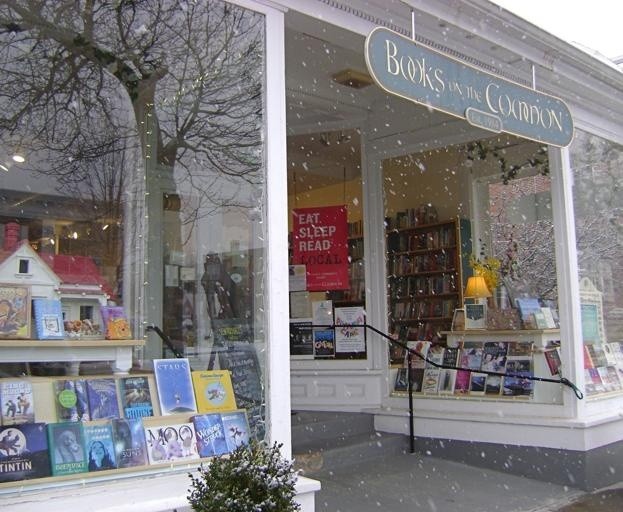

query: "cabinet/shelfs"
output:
385 219 471 364
287 236 364 307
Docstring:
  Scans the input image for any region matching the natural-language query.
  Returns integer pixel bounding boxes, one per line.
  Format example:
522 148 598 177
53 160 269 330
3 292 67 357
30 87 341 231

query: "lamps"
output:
330 69 373 89
465 276 491 304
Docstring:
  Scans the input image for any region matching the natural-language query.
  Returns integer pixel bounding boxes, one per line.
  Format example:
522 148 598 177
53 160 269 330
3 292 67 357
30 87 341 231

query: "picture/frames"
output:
1 282 32 339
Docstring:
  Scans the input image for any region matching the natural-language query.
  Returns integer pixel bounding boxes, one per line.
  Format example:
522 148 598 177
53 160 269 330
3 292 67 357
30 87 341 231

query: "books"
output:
344 261 365 301
393 323 443 362
192 369 237 414
0 380 35 425
152 358 197 416
393 300 456 318
349 220 362 237
397 339 536 399
86 378 120 421
398 226 456 252
99 306 130 339
83 423 117 471
221 412 250 452
0 423 50 483
584 343 623 395
517 299 554 328
390 252 454 271
55 379 90 422
545 350 561 375
146 423 201 464
35 299 65 339
395 203 437 228
390 274 451 297
119 376 154 419
191 413 228 456
349 240 364 258
110 417 148 467
47 421 90 476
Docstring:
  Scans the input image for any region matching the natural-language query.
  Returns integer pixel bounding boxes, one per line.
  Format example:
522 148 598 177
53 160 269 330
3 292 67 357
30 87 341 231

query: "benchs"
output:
1 470 320 512
